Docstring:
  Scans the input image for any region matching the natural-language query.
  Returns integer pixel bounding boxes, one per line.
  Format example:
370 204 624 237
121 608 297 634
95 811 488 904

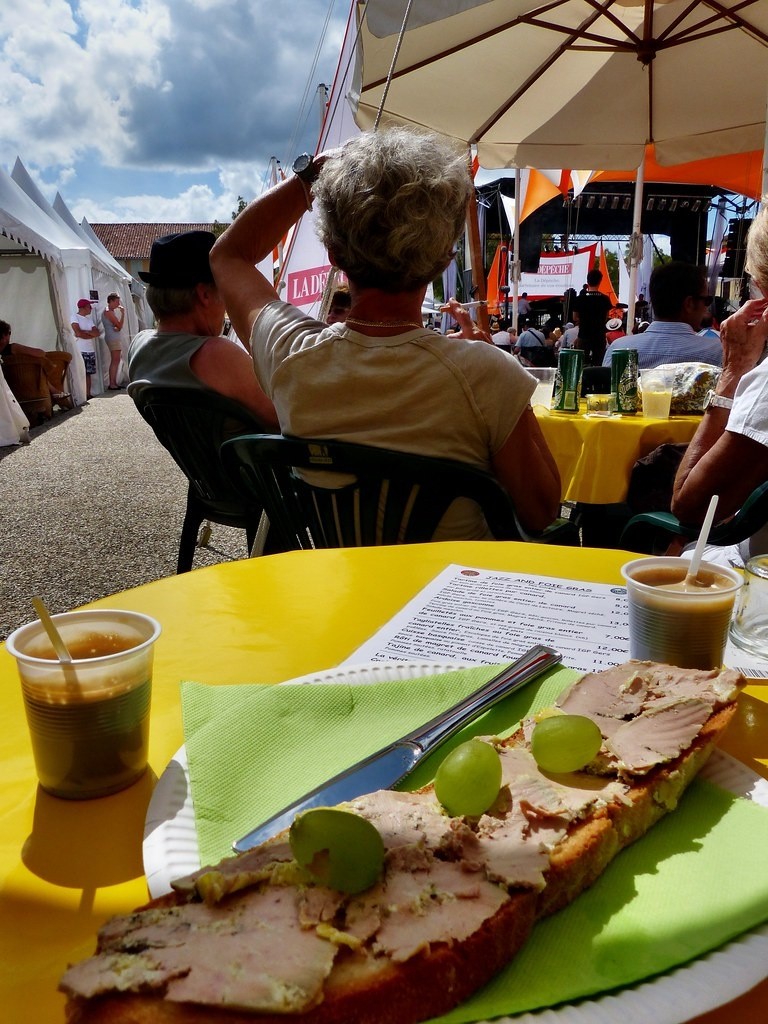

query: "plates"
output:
141 662 768 1022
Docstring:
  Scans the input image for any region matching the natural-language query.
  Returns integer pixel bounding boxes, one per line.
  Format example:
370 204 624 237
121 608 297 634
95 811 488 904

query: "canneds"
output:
611 348 638 416
553 349 584 414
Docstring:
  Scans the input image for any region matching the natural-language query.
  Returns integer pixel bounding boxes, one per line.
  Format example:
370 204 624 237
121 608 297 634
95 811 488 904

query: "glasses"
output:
693 295 714 307
327 307 347 315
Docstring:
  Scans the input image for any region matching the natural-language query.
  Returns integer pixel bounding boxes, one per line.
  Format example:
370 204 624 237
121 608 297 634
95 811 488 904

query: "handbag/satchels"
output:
532 347 558 367
580 365 611 398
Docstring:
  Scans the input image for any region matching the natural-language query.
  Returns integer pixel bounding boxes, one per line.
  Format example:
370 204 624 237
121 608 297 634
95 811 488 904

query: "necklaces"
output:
344 316 423 329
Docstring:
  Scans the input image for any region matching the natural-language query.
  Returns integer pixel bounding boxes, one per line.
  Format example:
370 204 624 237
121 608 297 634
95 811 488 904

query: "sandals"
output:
52 391 71 401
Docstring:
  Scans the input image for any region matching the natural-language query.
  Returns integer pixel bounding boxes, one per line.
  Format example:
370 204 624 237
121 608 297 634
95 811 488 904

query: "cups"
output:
523 366 558 416
639 368 676 420
620 557 742 670
5 608 162 800
729 553 768 662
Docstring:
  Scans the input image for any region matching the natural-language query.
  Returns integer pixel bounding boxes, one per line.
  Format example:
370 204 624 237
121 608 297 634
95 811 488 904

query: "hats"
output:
137 230 216 289
639 361 723 415
490 322 500 331
78 300 94 307
562 322 574 329
605 318 622 330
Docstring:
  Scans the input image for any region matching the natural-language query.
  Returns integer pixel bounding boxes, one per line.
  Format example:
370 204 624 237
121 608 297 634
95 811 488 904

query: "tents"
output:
0 156 155 444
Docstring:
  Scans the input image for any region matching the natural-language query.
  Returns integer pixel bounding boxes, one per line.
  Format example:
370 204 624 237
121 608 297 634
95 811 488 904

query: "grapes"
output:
530 715 604 774
433 739 503 819
289 809 385 893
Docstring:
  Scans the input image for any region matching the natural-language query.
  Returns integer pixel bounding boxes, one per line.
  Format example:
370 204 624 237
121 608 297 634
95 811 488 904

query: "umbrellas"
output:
344 0 768 333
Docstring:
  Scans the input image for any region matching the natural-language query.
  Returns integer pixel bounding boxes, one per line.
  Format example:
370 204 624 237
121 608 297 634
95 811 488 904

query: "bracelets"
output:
297 172 314 212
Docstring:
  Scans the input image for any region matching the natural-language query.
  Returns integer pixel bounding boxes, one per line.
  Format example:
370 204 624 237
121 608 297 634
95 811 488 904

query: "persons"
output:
71 299 100 399
317 262 752 369
125 230 304 558
210 130 560 549
593 205 767 558
0 320 73 412
101 292 125 391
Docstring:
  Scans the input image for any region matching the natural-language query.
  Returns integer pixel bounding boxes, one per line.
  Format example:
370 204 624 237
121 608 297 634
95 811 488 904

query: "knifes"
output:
232 645 562 854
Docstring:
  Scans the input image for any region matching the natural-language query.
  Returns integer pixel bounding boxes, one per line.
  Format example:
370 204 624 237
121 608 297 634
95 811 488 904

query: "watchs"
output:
702 390 734 410
291 153 320 183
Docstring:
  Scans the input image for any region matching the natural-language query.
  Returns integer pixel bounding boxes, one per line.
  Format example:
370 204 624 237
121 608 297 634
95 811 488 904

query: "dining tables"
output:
0 540 768 1024
529 393 702 525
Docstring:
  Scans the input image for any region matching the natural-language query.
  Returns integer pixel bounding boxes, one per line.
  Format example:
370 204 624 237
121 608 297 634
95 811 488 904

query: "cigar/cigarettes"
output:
440 300 489 312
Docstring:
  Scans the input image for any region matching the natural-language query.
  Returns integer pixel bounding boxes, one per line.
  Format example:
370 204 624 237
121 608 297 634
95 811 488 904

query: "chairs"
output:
520 345 556 367
496 344 511 355
615 481 768 553
216 432 577 549
581 367 613 398
125 380 268 575
1 351 73 423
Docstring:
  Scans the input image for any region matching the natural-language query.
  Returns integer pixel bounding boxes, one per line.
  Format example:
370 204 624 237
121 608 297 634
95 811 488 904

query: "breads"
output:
59 677 746 1024
